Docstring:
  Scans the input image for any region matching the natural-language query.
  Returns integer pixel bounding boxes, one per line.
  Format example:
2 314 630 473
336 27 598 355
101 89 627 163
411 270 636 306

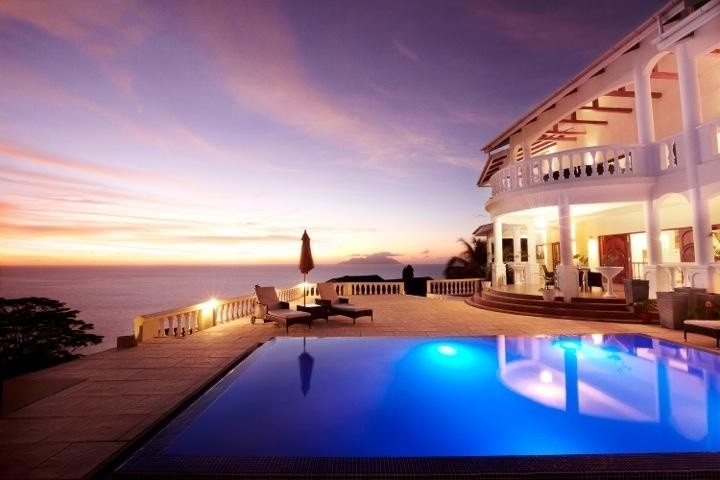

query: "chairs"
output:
250 284 311 333
541 264 554 287
315 282 373 325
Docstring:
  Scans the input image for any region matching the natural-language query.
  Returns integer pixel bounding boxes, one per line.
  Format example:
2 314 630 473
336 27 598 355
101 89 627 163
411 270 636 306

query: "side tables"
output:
297 304 329 325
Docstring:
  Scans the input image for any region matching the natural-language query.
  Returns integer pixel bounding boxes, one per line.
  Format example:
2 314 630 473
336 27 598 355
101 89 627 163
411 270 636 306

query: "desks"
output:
579 271 604 293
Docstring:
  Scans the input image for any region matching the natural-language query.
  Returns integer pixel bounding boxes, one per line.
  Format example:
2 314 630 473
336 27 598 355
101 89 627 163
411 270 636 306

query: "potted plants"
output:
538 273 562 301
632 295 655 323
479 266 495 289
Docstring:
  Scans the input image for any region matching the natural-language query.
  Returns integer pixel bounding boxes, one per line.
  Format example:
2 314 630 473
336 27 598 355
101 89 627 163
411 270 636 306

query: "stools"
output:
683 319 720 347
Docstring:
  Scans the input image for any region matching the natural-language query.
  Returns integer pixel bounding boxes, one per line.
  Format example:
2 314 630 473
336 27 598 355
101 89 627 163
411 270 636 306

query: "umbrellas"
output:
299 229 314 308
299 337 315 396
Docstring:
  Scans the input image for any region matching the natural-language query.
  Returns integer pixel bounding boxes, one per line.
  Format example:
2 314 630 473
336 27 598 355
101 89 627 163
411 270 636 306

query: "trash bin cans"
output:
624 280 648 303
657 287 719 329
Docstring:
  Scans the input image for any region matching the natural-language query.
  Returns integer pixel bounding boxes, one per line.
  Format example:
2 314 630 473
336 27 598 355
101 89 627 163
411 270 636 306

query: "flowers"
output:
573 253 589 267
